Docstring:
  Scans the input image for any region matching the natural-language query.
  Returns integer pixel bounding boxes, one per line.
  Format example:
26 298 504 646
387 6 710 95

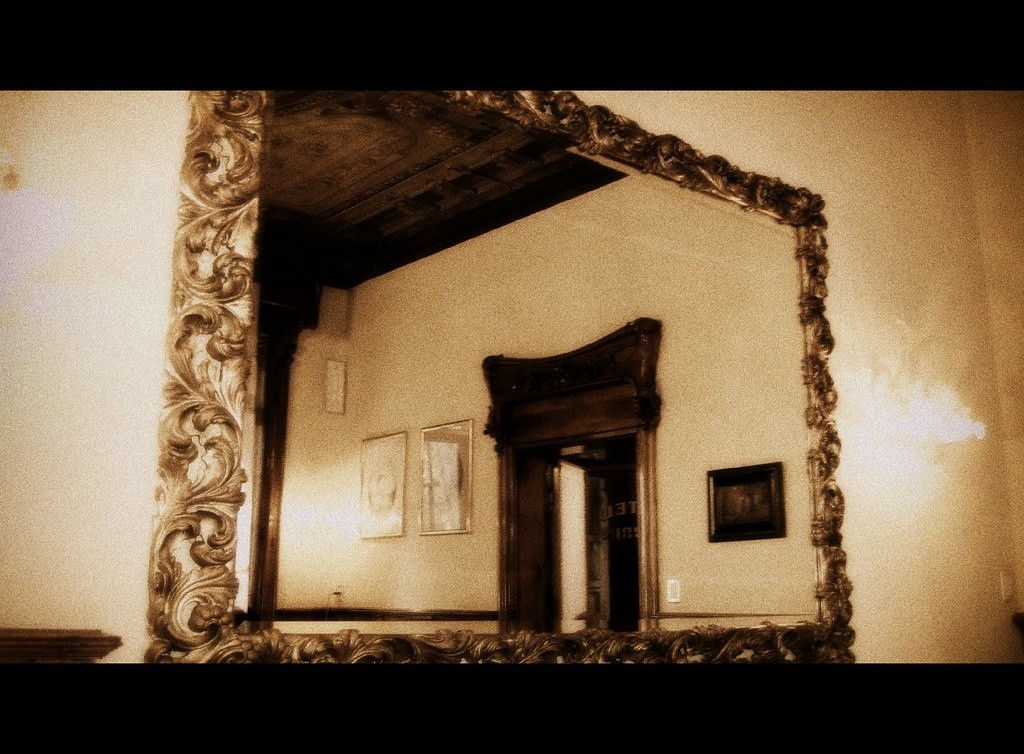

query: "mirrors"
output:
145 90 858 666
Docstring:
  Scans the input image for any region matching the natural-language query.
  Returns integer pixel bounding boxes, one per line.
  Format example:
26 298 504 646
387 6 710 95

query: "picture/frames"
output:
420 418 473 535
707 461 787 542
357 431 407 540
